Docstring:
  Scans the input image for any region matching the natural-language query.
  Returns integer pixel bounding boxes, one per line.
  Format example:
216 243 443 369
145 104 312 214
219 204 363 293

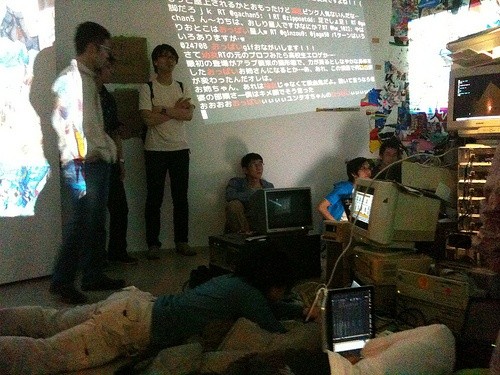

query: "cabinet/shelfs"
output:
457 146 496 233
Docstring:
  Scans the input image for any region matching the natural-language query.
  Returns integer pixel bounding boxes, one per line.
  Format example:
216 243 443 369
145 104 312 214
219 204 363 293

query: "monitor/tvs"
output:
322 285 375 354
447 63 500 134
257 186 314 234
399 161 459 215
345 177 442 251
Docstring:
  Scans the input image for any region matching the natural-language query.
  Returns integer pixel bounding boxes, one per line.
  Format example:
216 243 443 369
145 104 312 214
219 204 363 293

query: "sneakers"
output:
147 245 162 258
102 262 114 272
175 241 196 255
49 281 87 305
107 256 139 265
82 277 125 291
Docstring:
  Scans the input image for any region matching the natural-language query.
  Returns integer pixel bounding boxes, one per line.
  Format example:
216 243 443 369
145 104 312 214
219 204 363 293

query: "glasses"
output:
85 42 113 53
160 54 177 61
359 166 373 171
249 161 265 168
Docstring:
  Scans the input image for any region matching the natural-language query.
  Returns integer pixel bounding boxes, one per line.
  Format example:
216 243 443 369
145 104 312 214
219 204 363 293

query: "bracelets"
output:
161 108 166 115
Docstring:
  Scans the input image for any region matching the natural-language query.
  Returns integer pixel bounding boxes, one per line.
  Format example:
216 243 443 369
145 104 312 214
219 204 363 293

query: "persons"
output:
217 323 496 375
373 139 403 183
50 20 139 304
318 157 373 222
138 44 197 259
224 153 274 234
1 250 294 375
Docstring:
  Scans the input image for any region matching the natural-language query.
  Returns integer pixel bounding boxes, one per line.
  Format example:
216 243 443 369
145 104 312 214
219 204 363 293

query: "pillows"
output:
148 317 321 375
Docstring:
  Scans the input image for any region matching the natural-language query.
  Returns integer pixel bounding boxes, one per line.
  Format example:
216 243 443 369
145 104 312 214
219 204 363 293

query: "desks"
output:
209 233 320 285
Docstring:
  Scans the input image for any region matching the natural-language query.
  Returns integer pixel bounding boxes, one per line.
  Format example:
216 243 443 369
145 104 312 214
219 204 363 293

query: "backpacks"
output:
138 81 184 147
